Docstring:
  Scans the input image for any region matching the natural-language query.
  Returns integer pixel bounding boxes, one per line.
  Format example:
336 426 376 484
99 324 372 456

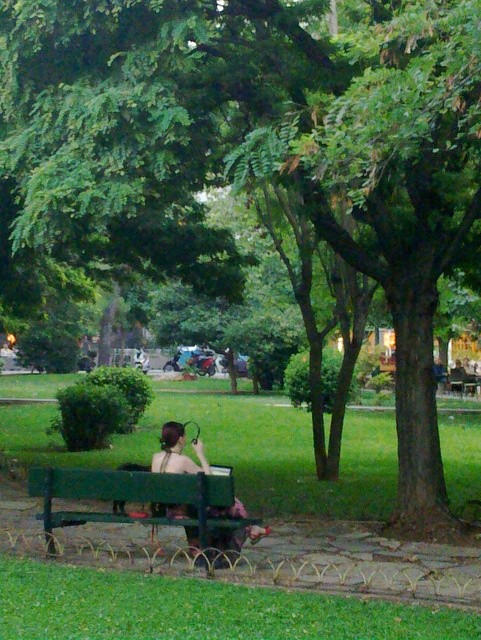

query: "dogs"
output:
113 462 180 519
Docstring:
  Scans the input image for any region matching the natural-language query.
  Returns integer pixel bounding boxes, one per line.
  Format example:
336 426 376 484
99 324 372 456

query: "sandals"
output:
250 527 272 544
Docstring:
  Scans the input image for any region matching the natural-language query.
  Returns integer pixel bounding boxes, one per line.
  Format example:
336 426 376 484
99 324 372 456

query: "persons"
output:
150 420 273 545
431 356 478 397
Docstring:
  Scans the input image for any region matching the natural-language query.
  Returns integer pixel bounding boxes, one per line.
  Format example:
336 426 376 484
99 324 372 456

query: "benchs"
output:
26 465 264 566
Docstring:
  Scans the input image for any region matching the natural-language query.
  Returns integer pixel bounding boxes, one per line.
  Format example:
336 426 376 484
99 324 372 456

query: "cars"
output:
112 348 150 375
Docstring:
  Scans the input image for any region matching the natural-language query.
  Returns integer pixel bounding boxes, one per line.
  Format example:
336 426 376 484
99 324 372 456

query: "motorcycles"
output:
164 349 187 376
186 345 216 378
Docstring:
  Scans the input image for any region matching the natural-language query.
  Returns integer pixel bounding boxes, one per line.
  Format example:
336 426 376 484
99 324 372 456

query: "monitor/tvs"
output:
208 464 232 476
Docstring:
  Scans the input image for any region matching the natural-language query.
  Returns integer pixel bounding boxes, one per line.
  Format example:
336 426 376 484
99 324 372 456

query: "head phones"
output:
174 413 202 450
